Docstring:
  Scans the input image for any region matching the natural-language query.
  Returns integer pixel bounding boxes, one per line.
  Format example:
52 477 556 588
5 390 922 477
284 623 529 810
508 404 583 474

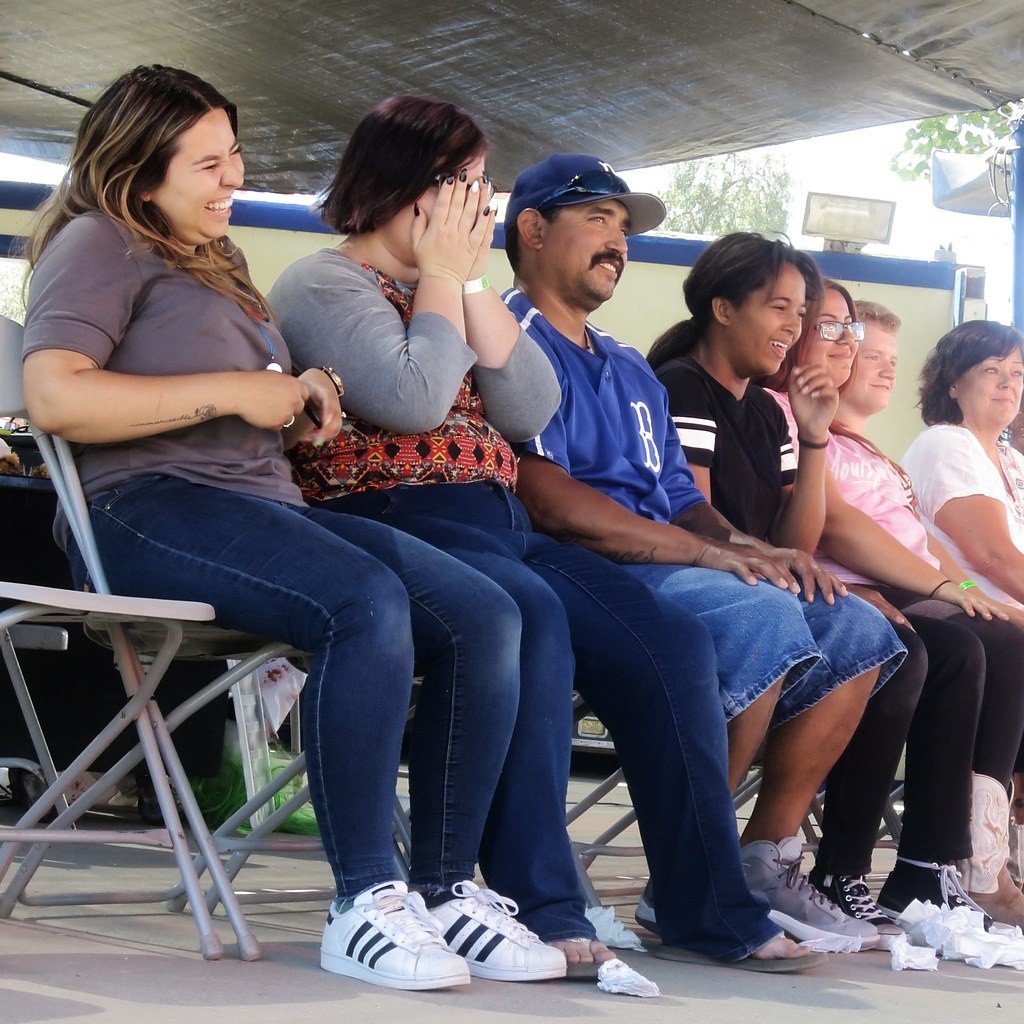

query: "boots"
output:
956 770 1024 931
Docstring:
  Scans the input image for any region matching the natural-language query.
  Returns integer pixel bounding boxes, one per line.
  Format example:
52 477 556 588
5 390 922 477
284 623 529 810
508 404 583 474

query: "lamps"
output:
802 191 897 254
930 135 1017 219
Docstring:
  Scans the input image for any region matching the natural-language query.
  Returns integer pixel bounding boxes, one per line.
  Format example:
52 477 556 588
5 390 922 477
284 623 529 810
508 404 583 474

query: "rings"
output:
341 412 347 417
283 415 294 428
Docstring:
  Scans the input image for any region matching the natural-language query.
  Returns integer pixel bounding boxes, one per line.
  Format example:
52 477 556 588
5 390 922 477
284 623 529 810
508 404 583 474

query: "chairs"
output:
0 311 906 963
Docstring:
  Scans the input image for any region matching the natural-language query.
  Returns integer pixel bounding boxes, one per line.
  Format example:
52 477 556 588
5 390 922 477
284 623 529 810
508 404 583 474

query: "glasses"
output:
815 321 867 342
434 172 496 201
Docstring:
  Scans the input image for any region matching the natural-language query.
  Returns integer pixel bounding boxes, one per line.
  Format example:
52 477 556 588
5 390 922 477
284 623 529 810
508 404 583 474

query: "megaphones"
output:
930 148 1015 219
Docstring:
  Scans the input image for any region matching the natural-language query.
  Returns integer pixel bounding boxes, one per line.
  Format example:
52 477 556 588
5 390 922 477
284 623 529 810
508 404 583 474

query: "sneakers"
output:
875 860 994 935
743 836 880 951
636 880 773 931
808 863 909 948
319 878 471 988
429 876 568 984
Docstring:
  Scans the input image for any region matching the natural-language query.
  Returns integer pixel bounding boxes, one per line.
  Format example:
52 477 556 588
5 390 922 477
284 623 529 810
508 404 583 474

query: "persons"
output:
644 233 1002 961
498 154 911 955
769 275 1024 893
263 92 820 979
15 64 568 989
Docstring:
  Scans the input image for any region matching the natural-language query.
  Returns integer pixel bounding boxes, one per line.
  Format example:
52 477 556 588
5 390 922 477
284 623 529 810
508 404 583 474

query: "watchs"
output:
322 365 345 398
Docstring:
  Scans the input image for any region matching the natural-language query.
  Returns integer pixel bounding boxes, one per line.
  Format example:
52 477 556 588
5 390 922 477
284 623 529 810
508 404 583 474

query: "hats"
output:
504 151 668 234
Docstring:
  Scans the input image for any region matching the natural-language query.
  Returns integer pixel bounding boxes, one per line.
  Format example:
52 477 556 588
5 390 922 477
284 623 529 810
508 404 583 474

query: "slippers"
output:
541 934 618 979
652 932 831 974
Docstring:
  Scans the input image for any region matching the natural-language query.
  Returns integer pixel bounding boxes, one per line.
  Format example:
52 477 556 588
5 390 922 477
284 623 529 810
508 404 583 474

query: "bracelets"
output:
462 274 490 296
959 581 978 590
929 580 951 599
797 432 829 449
